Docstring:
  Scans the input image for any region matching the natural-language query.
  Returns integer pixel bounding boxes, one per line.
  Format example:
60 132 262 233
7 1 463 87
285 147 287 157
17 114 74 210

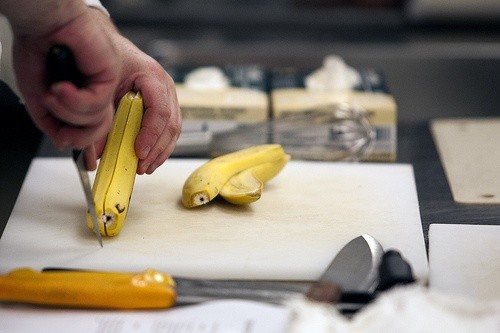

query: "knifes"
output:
40 44 104 250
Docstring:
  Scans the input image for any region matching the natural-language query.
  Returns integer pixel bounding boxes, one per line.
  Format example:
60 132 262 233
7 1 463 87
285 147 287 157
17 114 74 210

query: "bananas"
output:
183 143 289 209
85 91 143 239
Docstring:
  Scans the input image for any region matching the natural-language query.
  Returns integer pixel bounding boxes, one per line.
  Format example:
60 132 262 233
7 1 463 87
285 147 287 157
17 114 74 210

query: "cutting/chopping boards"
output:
0 155 431 333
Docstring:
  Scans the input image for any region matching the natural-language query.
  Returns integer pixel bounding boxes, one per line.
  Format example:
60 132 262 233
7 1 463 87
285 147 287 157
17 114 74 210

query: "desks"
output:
0 102 500 332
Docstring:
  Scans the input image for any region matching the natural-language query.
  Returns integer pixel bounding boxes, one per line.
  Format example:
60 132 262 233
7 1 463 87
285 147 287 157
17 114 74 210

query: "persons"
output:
0 0 183 175
